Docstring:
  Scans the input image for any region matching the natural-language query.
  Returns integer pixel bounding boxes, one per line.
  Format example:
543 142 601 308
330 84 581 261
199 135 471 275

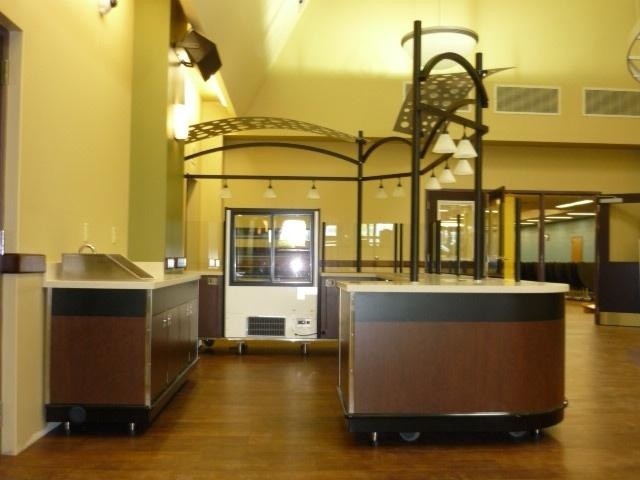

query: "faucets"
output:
78 244 95 254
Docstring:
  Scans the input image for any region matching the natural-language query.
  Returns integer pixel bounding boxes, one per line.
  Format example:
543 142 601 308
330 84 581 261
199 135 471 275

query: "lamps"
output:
401 26 479 72
183 73 477 200
176 32 222 81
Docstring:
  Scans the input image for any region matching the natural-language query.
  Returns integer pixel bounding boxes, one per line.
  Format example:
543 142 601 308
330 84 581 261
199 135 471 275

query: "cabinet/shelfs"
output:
321 277 379 340
39 279 200 416
197 274 224 338
